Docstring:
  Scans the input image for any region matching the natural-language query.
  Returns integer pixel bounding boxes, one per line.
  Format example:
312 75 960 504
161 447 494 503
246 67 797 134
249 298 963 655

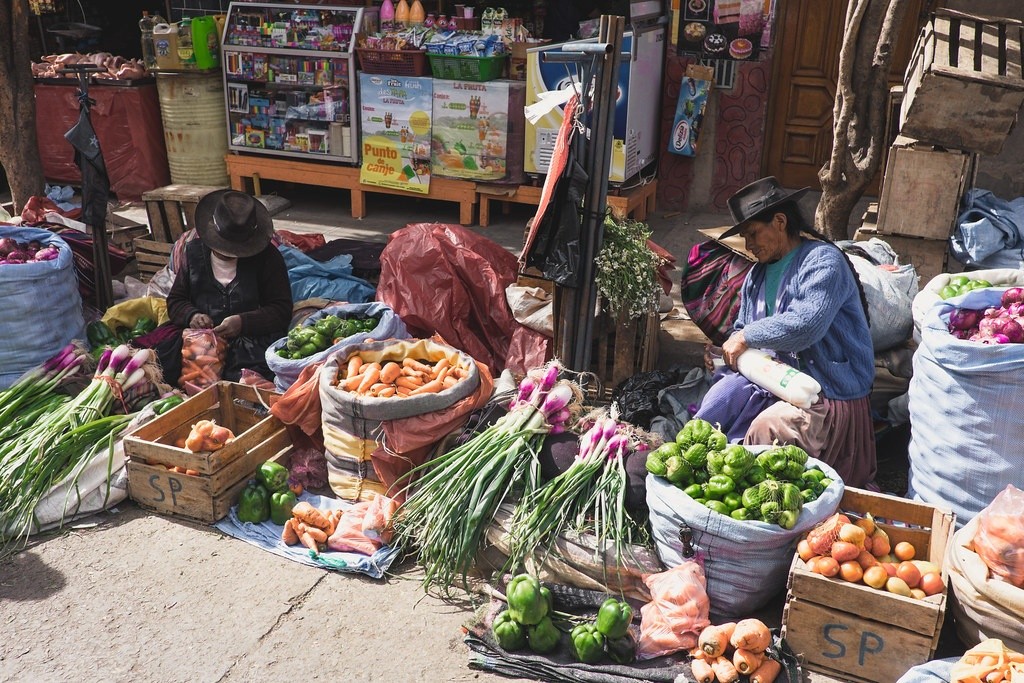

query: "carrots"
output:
282 495 401 555
951 639 1024 683
974 514 1024 586
639 559 781 683
334 356 467 398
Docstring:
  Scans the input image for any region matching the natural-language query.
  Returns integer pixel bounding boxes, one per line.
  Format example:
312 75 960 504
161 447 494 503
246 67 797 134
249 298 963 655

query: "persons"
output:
148 188 294 383
694 177 880 489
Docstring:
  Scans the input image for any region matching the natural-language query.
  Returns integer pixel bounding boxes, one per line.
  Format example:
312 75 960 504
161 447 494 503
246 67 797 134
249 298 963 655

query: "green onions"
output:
1 342 177 559
384 358 659 603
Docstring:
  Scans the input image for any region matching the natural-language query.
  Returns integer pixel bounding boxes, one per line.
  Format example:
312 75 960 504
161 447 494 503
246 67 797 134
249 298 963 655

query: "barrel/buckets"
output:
191 14 227 70
151 71 228 188
153 23 179 68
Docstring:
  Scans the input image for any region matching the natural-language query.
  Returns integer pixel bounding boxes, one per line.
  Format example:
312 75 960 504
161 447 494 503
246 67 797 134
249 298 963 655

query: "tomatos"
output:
797 513 943 600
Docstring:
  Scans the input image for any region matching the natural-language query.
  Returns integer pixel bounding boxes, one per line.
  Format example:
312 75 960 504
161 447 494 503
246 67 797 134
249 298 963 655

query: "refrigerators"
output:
524 23 668 196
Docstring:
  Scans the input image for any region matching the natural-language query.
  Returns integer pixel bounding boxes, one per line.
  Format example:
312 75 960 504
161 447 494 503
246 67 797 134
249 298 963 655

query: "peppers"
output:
238 485 270 523
270 490 297 525
275 315 376 361
256 461 289 491
646 420 831 528
87 318 156 362
940 279 994 301
493 573 637 666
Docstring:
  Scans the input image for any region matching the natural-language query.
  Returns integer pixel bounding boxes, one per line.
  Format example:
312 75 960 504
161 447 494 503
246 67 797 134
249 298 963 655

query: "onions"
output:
0 238 57 264
948 287 1024 346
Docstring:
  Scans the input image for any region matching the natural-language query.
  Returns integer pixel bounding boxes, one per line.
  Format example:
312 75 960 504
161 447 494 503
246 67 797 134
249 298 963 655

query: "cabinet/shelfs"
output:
219 1 381 167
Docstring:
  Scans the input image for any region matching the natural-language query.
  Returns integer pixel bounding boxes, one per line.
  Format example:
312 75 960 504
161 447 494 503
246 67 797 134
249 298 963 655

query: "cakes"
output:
684 23 706 41
729 38 752 59
703 34 728 55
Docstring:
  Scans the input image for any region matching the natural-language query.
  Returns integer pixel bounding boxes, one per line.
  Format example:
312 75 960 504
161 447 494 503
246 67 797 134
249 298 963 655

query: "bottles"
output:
320 41 344 51
409 0 425 25
385 111 392 128
424 12 436 27
176 15 195 69
478 116 490 140
737 348 822 410
449 13 455 28
229 27 279 47
436 13 448 28
394 0 409 27
138 11 155 69
469 94 482 120
400 126 409 143
379 0 394 28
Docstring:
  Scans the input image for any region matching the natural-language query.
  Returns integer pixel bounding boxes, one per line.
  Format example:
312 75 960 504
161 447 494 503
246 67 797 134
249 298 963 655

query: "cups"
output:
709 347 726 375
454 5 465 17
462 7 475 18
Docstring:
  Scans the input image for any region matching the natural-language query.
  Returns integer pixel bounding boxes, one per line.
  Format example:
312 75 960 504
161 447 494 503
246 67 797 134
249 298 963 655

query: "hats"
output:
717 176 812 240
195 189 274 258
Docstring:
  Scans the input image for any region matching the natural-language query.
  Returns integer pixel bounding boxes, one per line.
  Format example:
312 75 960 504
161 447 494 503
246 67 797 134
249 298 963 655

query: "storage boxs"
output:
852 199 951 294
121 378 295 528
133 233 192 286
898 6 1024 157
509 37 553 82
142 185 231 244
874 133 971 241
79 209 149 259
779 485 958 683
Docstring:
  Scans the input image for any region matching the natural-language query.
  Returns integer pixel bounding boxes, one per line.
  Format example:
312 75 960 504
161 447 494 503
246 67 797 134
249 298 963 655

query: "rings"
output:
198 320 203 323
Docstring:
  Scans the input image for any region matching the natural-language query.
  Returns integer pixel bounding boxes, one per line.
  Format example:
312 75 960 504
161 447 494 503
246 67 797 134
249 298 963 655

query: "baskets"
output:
353 46 426 76
427 52 510 81
